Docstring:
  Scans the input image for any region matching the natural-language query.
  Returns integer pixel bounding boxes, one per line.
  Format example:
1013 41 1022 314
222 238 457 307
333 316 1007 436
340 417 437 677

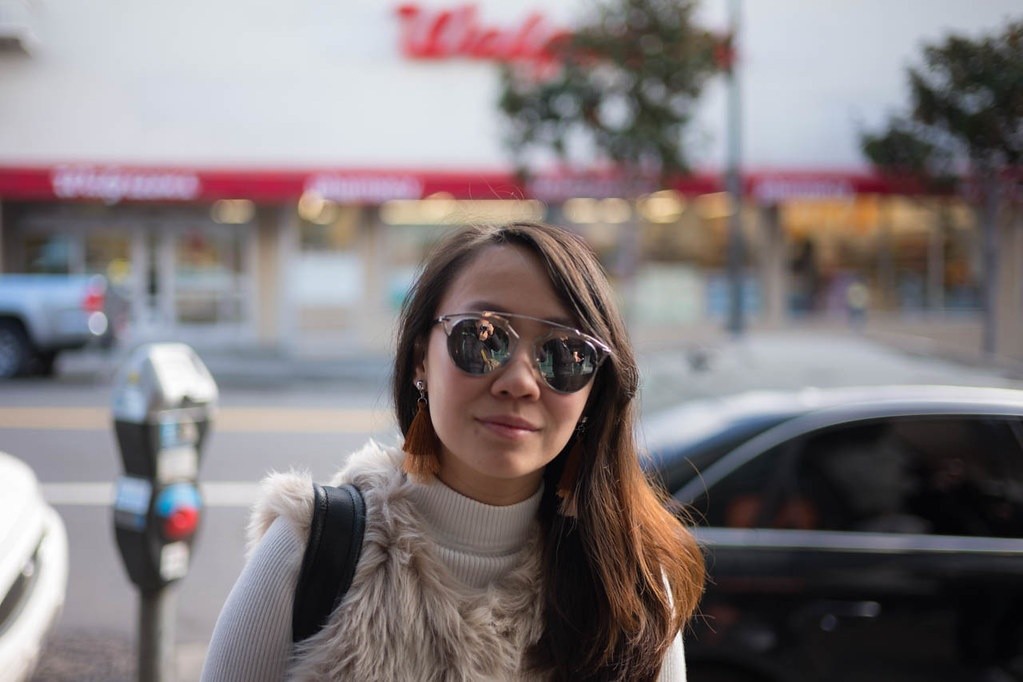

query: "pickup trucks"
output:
1 270 113 382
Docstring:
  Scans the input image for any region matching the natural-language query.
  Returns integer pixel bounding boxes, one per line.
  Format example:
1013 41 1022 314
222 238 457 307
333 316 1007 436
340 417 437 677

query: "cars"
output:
0 448 68 682
636 383 1023 682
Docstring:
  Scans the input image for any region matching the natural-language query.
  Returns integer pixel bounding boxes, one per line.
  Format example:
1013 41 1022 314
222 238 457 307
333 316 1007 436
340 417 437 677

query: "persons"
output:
197 220 709 682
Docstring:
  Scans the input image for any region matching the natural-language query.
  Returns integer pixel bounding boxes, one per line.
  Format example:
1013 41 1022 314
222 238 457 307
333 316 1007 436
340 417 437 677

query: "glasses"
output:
431 311 610 395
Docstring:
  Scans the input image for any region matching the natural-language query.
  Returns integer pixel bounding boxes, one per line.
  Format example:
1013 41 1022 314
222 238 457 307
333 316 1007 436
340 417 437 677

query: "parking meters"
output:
110 339 221 682
